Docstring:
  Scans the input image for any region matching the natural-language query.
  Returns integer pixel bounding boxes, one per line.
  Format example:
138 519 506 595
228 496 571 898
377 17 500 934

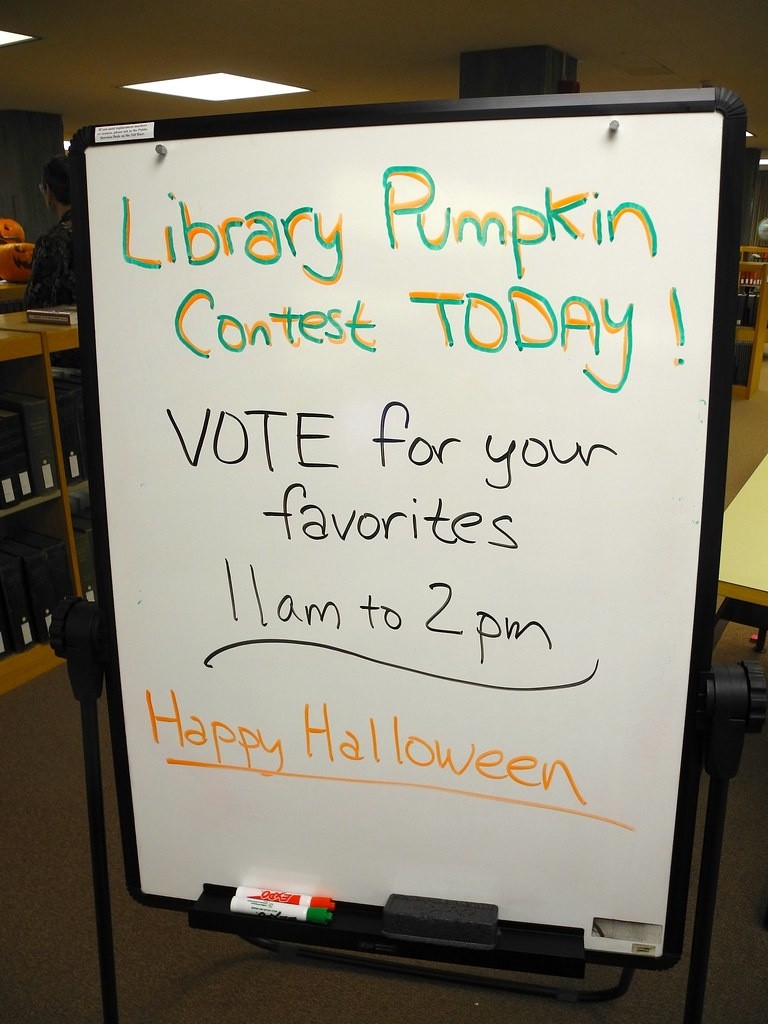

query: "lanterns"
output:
0 242 36 284
0 216 25 244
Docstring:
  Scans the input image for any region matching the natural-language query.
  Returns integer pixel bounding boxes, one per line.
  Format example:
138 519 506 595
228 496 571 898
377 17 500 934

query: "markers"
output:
230 886 336 922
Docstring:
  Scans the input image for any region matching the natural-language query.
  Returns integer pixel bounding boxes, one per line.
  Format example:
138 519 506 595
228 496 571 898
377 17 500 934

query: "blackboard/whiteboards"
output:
68 86 751 972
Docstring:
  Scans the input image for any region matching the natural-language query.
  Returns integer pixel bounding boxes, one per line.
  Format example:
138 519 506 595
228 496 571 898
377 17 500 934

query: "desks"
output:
716 452 768 654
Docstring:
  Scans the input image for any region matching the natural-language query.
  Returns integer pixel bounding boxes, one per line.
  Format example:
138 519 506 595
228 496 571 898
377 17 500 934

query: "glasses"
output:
39 183 46 194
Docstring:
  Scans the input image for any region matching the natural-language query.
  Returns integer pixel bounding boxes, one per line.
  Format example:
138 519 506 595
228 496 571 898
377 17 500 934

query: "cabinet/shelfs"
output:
732 262 768 400
1 305 100 697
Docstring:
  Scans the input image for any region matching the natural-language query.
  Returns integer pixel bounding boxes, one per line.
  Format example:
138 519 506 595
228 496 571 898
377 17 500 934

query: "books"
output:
736 287 759 327
0 366 88 510
732 341 753 387
0 509 96 661
26 304 78 325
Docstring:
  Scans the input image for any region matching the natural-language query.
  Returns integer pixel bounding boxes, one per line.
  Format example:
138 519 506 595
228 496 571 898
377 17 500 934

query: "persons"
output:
23 153 84 366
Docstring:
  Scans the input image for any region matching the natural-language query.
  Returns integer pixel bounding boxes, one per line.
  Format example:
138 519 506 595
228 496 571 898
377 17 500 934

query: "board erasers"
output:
380 894 499 950
